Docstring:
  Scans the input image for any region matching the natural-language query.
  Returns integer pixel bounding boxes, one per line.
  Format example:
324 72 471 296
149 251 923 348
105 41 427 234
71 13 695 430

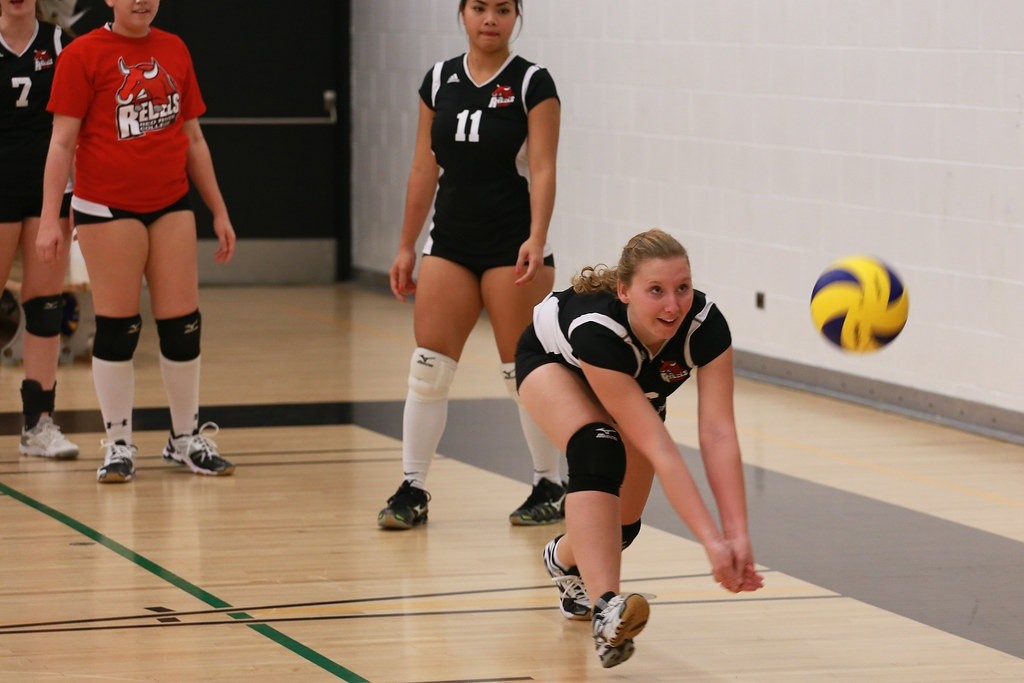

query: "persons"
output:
36 1 237 483
376 0 568 529
515 230 764 668
1 1 113 459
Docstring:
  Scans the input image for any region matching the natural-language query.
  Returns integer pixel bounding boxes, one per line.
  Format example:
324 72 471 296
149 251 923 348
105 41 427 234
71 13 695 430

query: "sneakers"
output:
539 532 594 620
589 590 651 668
376 479 432 529
161 421 237 477
508 476 569 526
17 416 80 460
96 438 139 484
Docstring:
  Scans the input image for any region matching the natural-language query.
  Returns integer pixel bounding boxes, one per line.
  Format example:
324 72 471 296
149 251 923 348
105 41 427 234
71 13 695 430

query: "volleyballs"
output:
810 254 910 353
